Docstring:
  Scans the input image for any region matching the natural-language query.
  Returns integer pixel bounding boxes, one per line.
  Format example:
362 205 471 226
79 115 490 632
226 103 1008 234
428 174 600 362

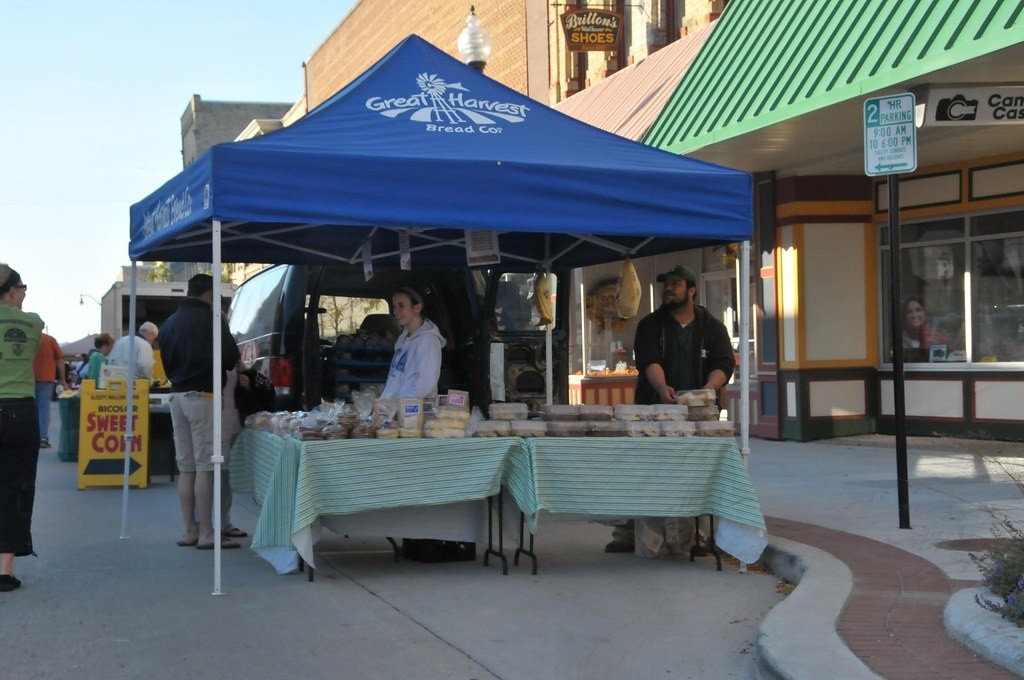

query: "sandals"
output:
221 528 248 536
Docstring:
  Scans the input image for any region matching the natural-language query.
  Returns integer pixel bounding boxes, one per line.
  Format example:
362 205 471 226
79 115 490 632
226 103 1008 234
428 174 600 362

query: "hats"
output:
189 275 226 305
656 267 697 281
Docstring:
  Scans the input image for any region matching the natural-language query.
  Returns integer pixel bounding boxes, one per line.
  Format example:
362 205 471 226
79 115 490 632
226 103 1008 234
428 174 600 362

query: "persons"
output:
76 322 160 391
160 274 247 548
375 283 446 562
0 261 43 591
903 297 950 347
33 321 66 448
595 264 736 556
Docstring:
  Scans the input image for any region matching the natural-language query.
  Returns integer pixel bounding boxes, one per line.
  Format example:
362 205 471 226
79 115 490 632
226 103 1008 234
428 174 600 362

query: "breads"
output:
242 408 469 441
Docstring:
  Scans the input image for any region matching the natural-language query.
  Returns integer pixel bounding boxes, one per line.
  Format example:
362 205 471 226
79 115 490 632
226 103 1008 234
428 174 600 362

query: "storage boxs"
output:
474 389 737 438
403 537 476 563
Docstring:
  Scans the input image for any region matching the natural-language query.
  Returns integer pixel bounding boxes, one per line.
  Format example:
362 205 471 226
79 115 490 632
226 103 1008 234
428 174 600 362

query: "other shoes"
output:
605 539 635 552
691 546 707 557
0 575 20 590
40 440 51 447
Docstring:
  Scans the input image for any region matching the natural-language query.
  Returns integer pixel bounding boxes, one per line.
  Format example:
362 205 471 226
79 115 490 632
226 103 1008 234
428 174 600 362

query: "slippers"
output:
197 537 240 548
177 535 197 546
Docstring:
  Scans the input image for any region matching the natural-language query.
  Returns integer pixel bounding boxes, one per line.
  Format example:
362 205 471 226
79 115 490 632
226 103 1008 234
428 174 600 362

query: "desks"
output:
229 429 536 582
514 437 769 576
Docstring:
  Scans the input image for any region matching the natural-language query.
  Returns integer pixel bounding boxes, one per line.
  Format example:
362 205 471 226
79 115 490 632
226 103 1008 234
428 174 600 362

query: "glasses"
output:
7 285 27 292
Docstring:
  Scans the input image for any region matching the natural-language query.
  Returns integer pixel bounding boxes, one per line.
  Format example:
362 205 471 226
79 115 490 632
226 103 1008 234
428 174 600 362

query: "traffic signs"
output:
863 93 917 176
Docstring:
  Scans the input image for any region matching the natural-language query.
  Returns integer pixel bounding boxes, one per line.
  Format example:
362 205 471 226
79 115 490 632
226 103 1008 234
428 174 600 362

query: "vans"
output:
226 264 572 414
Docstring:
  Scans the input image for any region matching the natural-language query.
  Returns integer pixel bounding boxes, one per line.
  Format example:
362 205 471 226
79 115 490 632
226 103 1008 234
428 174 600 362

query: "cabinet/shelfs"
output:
316 344 395 404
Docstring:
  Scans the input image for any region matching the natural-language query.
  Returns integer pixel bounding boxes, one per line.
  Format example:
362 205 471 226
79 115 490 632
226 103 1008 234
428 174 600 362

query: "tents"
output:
120 32 754 597
